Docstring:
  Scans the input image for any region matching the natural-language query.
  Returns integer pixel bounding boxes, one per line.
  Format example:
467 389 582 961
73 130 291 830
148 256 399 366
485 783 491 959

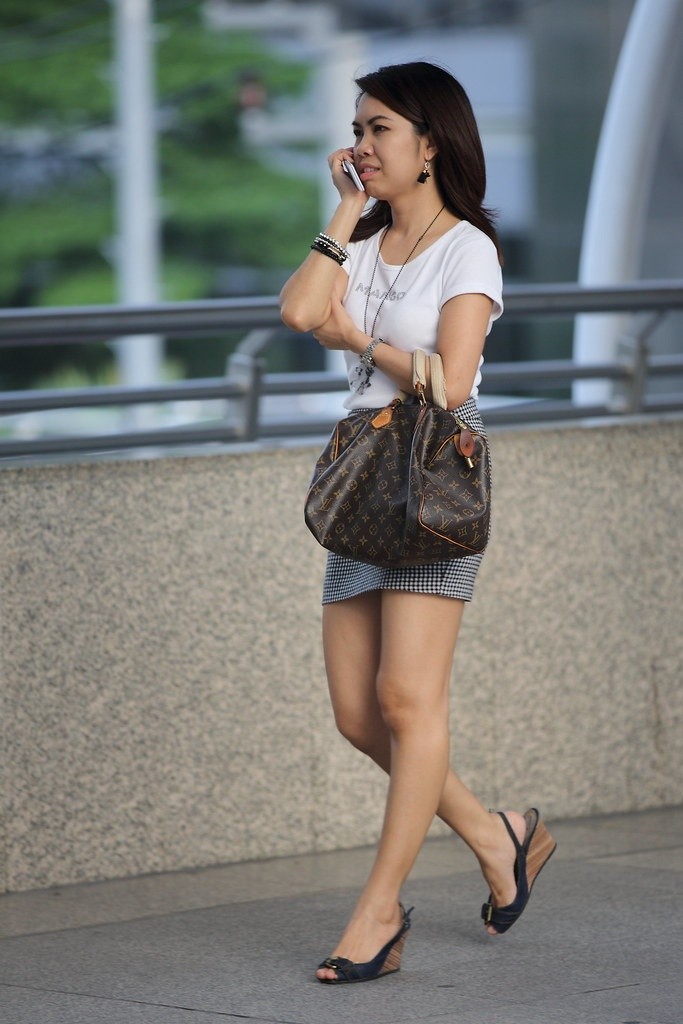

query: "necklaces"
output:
350 204 445 394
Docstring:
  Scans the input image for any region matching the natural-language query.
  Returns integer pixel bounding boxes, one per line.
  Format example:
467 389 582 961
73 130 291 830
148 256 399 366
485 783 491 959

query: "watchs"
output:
359 338 386 369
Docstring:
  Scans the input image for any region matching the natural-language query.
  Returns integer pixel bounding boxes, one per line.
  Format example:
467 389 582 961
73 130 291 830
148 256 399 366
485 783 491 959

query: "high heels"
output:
315 902 415 984
479 806 558 936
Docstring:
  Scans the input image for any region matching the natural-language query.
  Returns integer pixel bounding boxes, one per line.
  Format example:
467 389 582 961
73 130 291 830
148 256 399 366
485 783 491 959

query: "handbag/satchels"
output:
303 348 492 571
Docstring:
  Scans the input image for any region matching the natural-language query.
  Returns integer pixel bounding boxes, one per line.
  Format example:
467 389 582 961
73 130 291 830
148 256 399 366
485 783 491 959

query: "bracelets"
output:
310 231 350 265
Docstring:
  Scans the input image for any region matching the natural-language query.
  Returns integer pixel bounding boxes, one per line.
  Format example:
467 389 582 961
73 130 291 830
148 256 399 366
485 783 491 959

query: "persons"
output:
279 62 558 985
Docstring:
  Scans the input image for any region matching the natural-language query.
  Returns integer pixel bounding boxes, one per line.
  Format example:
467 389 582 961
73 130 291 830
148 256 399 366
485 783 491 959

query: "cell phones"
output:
344 159 365 192
